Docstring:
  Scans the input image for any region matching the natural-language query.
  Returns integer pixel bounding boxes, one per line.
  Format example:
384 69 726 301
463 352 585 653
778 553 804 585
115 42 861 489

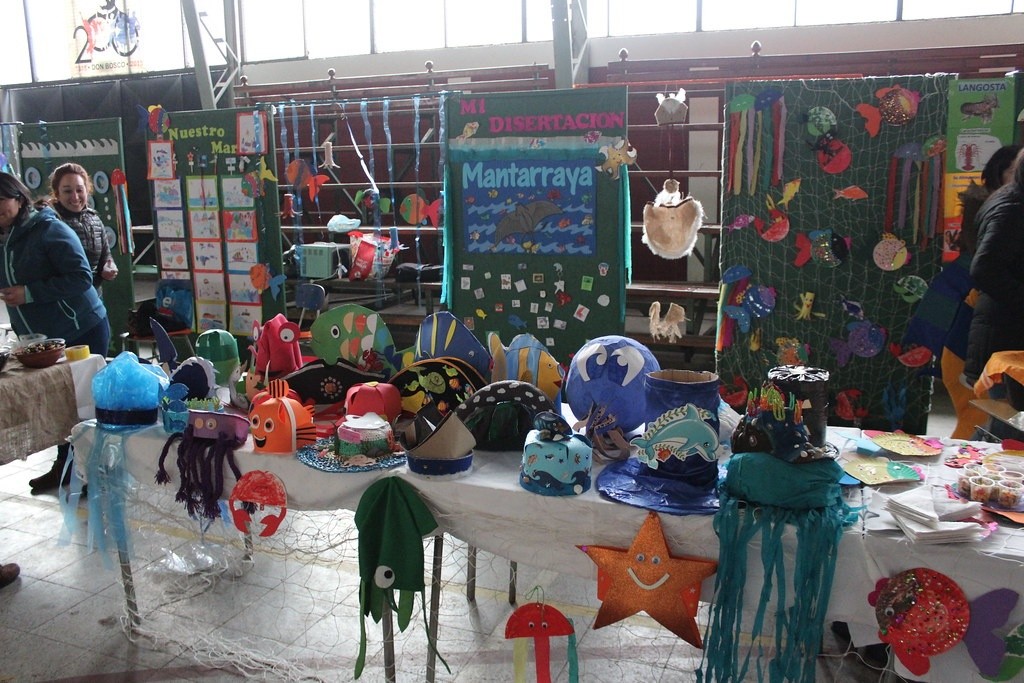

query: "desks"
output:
70 412 1024 683
1 347 109 463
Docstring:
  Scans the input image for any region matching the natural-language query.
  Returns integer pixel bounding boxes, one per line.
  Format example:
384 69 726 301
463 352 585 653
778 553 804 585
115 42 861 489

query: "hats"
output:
92 305 842 515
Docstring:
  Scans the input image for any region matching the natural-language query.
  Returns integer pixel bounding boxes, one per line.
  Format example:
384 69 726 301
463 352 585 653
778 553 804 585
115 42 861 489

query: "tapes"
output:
64 345 90 361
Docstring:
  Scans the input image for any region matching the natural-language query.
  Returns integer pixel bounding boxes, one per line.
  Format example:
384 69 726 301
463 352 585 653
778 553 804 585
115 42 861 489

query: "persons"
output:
959 143 1024 388
46 163 118 301
963 146 1024 438
0 172 110 502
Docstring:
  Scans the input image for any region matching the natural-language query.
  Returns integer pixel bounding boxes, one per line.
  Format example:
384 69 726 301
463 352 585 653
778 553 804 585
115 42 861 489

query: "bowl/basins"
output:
0 333 68 375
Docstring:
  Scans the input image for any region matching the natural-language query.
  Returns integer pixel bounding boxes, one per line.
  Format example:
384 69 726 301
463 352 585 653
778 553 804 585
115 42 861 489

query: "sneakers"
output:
28 460 74 488
0 562 22 587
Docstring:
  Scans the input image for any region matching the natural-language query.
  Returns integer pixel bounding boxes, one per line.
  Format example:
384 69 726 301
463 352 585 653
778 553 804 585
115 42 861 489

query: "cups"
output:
957 463 1024 510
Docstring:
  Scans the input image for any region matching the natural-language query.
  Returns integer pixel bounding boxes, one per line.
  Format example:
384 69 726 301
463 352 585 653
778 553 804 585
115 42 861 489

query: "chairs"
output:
120 277 197 362
